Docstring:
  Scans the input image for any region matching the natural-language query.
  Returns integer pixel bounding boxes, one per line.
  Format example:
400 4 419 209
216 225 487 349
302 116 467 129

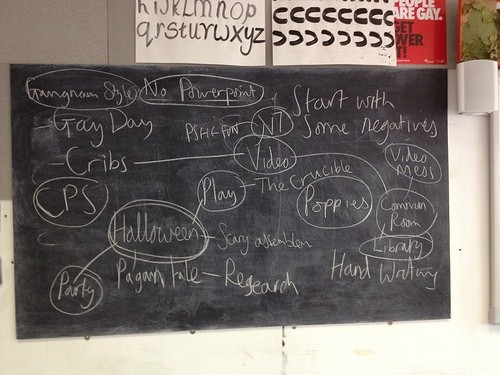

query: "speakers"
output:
457 59 499 115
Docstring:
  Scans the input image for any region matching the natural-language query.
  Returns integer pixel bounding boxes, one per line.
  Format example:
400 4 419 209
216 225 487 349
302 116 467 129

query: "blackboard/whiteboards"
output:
9 61 452 340
